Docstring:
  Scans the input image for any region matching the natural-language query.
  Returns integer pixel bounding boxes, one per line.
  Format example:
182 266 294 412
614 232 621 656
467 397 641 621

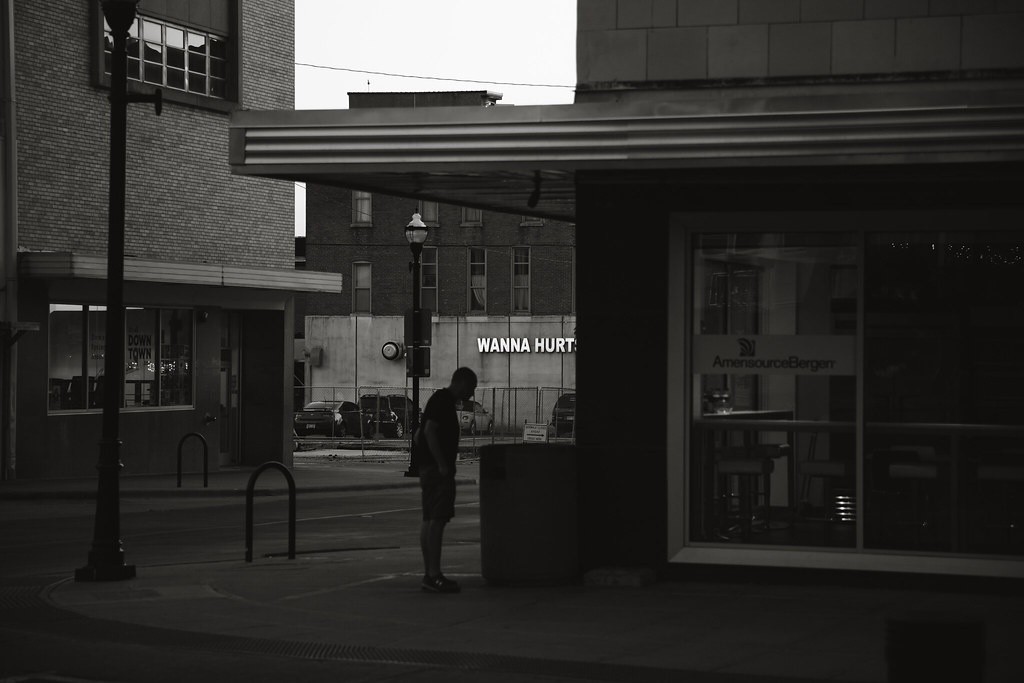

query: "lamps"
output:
199 310 210 321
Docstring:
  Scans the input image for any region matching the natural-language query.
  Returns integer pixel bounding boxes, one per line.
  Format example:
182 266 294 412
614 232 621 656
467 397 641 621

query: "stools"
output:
712 457 772 541
977 466 1024 544
878 463 938 550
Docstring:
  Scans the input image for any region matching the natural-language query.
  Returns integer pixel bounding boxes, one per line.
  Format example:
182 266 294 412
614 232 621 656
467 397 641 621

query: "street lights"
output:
71 0 138 582
403 208 432 476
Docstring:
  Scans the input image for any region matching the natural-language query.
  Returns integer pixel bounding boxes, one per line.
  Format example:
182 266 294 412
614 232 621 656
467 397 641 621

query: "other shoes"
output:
422 575 461 593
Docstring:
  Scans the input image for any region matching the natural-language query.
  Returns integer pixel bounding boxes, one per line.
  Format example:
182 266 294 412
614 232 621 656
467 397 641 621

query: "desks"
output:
707 406 795 522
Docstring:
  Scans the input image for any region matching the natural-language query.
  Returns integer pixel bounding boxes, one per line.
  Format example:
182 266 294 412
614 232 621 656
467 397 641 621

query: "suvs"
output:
356 393 423 439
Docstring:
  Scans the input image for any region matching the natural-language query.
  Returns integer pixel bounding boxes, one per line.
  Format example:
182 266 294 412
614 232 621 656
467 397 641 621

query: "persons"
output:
413 366 478 594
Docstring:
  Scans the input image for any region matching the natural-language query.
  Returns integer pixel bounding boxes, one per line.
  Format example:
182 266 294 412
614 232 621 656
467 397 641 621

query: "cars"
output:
551 392 575 430
292 399 377 441
456 400 493 437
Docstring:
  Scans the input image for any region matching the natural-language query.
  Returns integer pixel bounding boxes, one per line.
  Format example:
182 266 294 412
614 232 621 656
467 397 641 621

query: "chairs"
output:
797 460 848 513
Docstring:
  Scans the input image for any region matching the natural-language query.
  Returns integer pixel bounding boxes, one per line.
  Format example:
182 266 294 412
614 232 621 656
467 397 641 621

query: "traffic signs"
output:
523 422 549 444
